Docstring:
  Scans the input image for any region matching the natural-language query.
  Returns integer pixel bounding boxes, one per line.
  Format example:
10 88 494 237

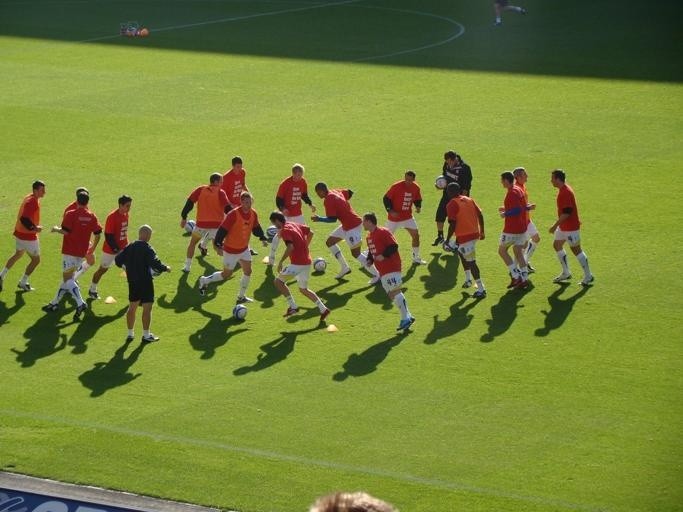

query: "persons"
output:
268 163 316 265
0 180 47 291
362 212 415 330
199 191 268 304
432 151 472 246
199 157 259 255
383 171 427 265
64 188 96 295
181 174 236 273
443 182 486 297
269 212 329 321
311 183 380 284
499 172 531 289
115 225 171 342
492 0 527 27
513 168 541 273
549 170 595 284
89 196 134 299
43 192 102 319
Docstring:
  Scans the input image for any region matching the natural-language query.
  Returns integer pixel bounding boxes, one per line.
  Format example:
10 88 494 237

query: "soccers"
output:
313 257 326 271
233 304 247 320
267 226 278 239
436 175 447 189
443 238 455 252
185 220 196 233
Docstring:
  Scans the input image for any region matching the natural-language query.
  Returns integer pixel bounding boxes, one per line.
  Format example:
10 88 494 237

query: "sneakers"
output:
527 263 536 273
432 237 446 246
250 249 258 255
319 309 330 321
127 334 134 340
462 279 472 288
283 306 299 317
553 272 572 283
181 267 191 273
198 275 207 297
368 271 381 284
404 316 415 330
521 280 529 289
578 274 594 284
88 288 101 300
18 280 35 290
412 256 427 265
335 265 351 279
142 334 160 342
42 302 58 312
73 302 88 321
507 276 522 288
198 242 210 256
237 295 253 302
396 318 413 332
469 290 486 298
